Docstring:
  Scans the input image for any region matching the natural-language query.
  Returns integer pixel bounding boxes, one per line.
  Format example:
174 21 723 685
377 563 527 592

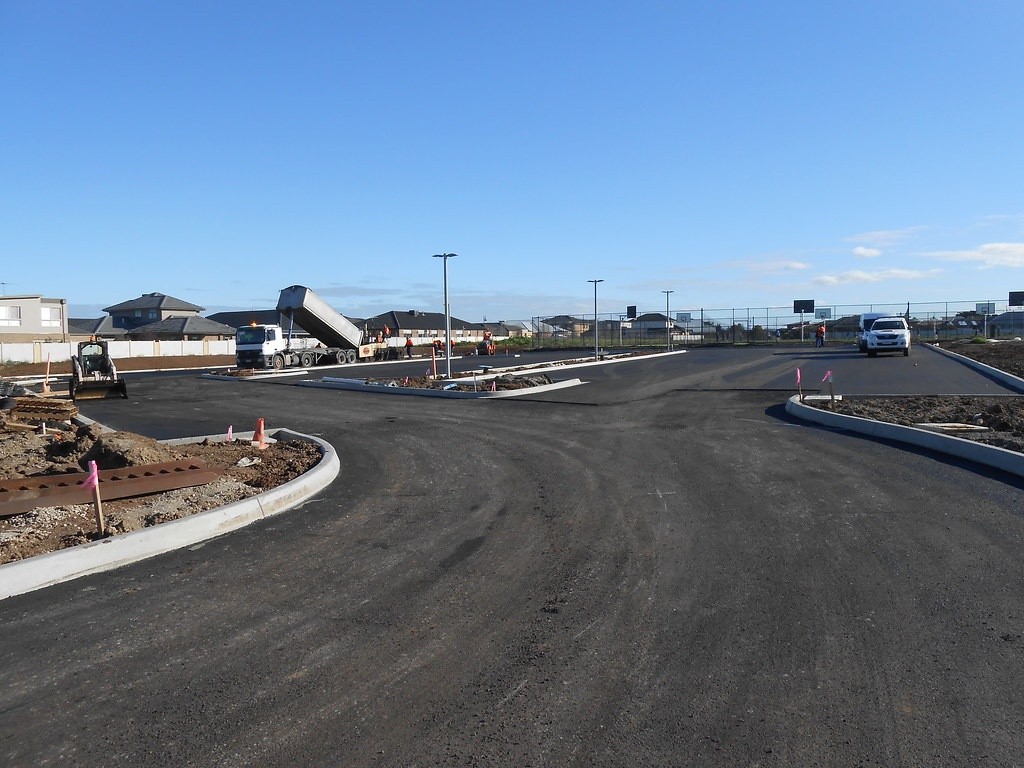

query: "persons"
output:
376 331 383 342
451 340 455 356
315 343 321 348
382 325 390 338
405 337 414 359
776 331 780 338
483 331 490 340
767 332 770 341
815 325 825 348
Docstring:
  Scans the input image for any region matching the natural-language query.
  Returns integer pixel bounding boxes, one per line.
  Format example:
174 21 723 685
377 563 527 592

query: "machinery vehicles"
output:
476 332 496 355
68 333 129 401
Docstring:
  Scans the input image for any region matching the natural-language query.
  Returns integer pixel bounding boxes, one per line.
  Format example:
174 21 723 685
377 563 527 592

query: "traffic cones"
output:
251 416 270 450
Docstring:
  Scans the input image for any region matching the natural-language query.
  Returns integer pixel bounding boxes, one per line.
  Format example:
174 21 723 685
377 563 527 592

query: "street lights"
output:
586 279 605 363
661 290 675 352
433 252 459 379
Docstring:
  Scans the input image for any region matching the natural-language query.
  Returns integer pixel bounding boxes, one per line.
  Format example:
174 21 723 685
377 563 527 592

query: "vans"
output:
857 311 898 354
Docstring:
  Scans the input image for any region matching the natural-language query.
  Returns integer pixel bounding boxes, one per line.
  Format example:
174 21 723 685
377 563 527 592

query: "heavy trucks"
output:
234 283 365 371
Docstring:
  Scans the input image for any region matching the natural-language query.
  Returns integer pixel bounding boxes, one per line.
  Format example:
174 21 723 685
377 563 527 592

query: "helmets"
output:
407 336 409 339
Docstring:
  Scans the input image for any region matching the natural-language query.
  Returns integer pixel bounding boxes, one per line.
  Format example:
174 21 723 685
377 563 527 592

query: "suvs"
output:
865 317 913 357
